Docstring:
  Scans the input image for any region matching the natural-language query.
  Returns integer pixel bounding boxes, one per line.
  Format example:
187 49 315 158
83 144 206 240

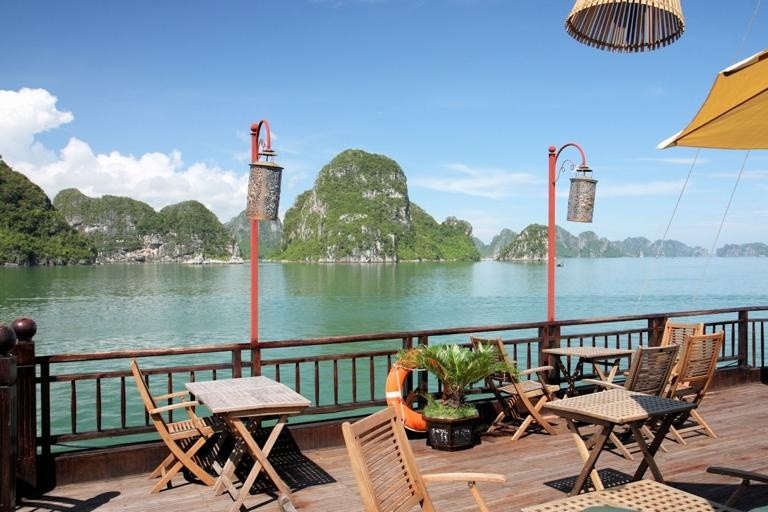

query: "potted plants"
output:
393 337 523 451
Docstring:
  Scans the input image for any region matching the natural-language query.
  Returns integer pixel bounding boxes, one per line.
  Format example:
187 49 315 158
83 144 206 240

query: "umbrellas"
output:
655 47 768 150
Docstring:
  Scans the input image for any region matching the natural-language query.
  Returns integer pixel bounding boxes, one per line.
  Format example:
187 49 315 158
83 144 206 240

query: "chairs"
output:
469 335 560 441
341 401 505 512
582 318 725 460
127 359 226 495
705 458 766 512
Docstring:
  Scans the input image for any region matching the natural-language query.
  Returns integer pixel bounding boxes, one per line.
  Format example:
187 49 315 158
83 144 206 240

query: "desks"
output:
541 389 696 497
520 479 747 512
184 376 311 512
540 345 632 430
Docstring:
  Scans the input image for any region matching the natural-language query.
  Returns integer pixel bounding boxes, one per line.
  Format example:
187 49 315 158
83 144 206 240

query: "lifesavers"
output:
385 349 460 433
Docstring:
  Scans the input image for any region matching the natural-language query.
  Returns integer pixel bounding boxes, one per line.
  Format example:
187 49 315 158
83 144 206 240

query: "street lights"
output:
246 118 284 341
548 144 598 320
